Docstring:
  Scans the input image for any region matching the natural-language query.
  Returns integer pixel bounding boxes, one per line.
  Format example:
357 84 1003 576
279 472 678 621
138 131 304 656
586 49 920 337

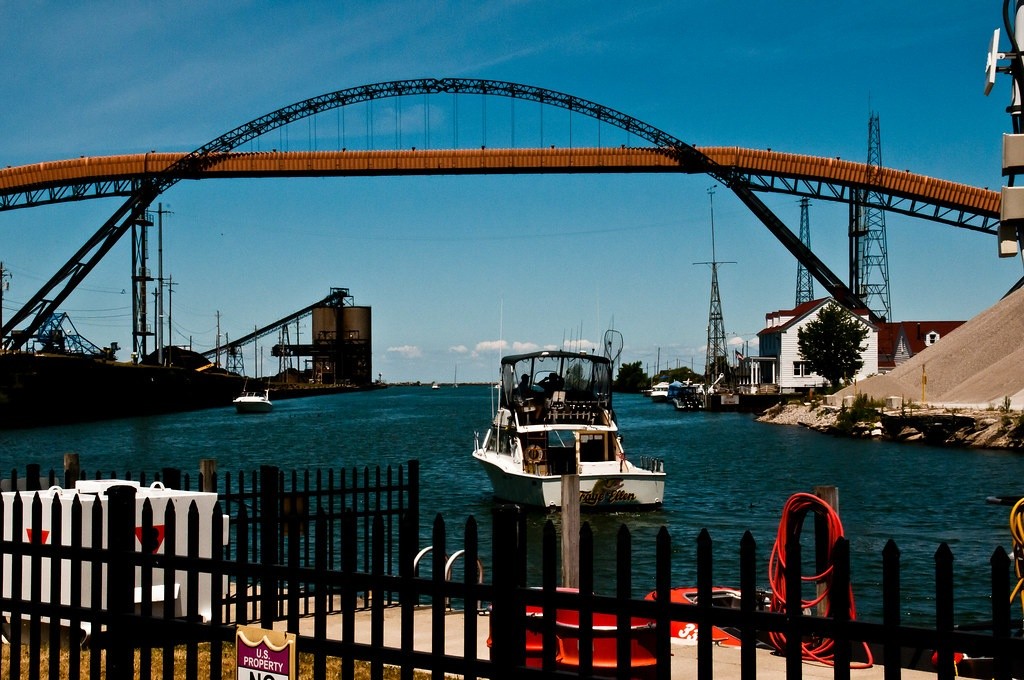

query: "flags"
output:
735 350 745 362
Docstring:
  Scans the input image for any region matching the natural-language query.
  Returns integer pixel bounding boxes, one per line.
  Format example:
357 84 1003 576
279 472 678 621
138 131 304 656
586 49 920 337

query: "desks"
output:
2 488 219 651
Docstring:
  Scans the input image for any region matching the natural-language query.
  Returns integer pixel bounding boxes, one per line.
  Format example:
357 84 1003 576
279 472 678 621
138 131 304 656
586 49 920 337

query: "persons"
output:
538 371 566 399
516 373 545 425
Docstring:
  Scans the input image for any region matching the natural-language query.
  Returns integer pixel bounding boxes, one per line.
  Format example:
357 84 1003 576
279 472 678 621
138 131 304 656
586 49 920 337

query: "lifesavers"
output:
524 444 543 464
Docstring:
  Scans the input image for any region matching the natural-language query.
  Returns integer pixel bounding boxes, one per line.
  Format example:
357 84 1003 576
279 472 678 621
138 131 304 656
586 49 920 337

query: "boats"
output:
232 392 273 414
641 382 703 412
473 349 667 514
642 584 780 649
